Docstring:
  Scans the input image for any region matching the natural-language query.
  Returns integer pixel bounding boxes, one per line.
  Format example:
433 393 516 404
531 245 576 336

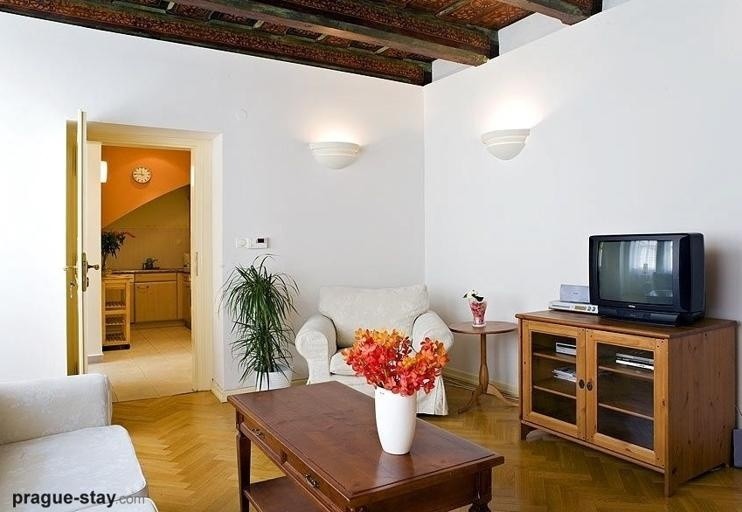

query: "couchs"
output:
0 373 158 512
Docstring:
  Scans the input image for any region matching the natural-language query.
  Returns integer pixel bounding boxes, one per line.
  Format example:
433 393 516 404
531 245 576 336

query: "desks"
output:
449 321 519 414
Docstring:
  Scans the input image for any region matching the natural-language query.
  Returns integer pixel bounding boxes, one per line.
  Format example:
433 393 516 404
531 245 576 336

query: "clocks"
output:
131 165 153 185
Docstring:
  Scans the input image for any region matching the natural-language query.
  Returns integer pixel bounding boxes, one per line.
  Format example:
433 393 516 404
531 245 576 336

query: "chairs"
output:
296 282 455 419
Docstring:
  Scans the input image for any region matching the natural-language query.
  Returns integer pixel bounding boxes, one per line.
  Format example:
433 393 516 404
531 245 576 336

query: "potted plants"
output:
211 250 304 391
101 229 135 277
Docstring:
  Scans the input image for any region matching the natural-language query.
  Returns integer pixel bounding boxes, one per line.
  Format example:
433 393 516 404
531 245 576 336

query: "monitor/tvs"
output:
588 233 704 328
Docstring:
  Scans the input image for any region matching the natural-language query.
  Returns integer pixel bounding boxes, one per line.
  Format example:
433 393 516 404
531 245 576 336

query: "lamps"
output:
309 141 361 171
480 127 531 161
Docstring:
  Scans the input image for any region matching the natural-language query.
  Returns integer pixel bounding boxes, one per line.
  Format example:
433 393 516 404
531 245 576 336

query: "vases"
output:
374 385 417 455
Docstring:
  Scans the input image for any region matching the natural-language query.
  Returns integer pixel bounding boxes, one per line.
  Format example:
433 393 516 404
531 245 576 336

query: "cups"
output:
469 293 487 328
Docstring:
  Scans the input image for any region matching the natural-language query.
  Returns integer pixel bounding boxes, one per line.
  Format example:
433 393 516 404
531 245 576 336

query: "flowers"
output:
339 326 451 401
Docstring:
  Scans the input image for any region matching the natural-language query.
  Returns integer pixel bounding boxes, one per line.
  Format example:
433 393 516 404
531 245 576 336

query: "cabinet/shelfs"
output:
102 271 184 352
515 310 741 499
